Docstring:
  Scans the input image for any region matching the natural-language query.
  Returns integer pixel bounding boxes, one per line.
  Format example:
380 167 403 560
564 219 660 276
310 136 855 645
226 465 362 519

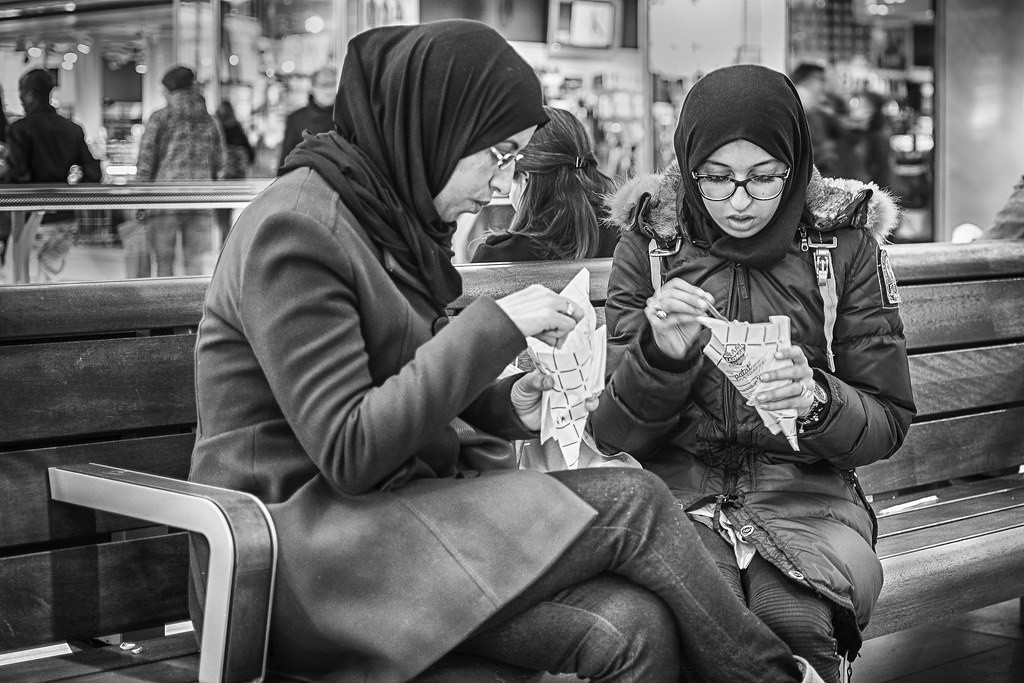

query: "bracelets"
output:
796 418 811 435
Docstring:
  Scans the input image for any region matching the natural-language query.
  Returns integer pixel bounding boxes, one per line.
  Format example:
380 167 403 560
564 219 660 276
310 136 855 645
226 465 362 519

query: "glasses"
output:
489 145 527 170
690 168 794 200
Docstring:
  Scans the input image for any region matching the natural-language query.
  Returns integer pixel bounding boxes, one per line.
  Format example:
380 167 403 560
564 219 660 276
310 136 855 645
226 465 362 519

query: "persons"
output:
214 100 255 164
275 67 339 175
133 65 222 276
789 65 892 195
117 204 151 279
188 20 828 683
466 103 624 262
599 64 917 683
0 60 102 283
972 176 1024 243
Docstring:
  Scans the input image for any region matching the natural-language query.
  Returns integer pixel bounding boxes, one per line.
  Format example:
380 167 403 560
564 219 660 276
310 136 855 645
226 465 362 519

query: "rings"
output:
655 302 666 320
566 299 574 317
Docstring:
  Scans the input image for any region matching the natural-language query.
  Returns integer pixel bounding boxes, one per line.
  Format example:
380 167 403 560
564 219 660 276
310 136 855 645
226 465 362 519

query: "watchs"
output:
795 380 828 416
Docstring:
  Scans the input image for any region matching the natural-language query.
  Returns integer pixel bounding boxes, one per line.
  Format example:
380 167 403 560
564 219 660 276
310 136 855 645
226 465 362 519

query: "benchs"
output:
0 239 1024 683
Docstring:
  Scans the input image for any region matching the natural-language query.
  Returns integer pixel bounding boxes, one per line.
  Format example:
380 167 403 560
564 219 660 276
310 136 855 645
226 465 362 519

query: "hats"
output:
160 67 195 92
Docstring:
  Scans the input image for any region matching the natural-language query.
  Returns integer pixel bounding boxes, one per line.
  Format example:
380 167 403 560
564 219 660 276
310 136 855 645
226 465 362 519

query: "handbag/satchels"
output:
118 223 153 279
